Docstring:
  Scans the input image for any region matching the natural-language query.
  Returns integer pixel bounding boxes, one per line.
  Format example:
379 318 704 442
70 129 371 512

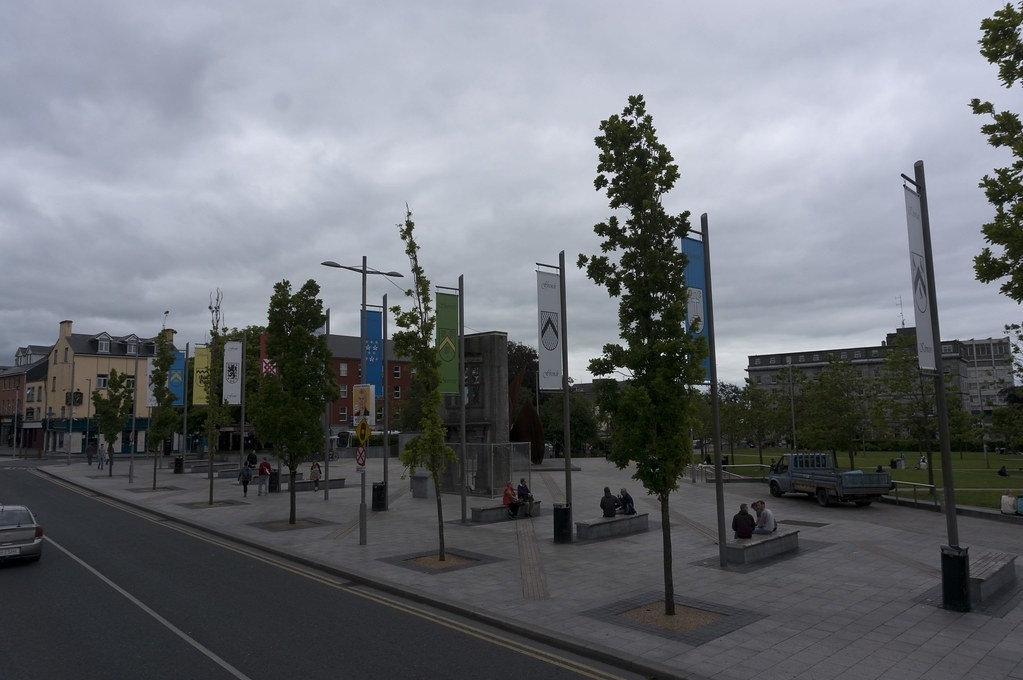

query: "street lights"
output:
85 378 90 459
322 257 406 542
13 385 22 456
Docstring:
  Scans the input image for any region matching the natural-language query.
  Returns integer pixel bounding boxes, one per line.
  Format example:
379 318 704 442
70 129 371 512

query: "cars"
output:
0 505 44 559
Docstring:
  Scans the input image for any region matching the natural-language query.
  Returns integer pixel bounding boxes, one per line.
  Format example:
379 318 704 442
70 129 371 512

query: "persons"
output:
616 488 633 514
86 442 95 465
311 460 321 492
704 454 712 465
258 457 271 496
732 504 755 539
600 487 620 517
503 481 519 519
237 461 252 497
751 501 777 534
1001 490 1023 516
247 449 257 467
517 478 535 517
722 456 727 470
98 443 106 469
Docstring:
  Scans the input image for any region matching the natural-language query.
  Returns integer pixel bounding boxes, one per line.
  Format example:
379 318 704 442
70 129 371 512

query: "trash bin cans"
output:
269 468 279 493
896 458 905 469
940 544 971 612
553 502 571 543
174 457 183 474
412 470 432 498
372 481 385 511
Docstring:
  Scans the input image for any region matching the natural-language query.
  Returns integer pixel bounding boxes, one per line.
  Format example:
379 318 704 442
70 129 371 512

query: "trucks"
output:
771 453 893 508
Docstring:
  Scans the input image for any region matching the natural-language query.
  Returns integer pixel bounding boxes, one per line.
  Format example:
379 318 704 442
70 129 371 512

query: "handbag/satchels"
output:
524 493 535 502
263 466 270 476
622 500 636 516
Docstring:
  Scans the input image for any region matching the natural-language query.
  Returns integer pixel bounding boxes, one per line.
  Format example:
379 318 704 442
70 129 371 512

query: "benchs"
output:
574 513 649 540
726 526 800 564
969 551 1018 605
470 500 541 523
168 454 346 491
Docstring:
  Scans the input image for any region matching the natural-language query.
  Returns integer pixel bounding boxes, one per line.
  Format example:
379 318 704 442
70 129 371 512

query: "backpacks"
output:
242 468 252 479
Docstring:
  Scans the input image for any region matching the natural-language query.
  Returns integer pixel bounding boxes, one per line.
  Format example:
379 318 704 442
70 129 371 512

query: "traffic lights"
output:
11 413 15 424
16 413 24 428
47 418 54 431
41 418 47 432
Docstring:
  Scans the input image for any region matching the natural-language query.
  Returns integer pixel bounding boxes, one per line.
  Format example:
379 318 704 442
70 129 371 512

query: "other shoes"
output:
528 514 534 518
508 514 515 520
525 514 530 518
508 509 513 515
